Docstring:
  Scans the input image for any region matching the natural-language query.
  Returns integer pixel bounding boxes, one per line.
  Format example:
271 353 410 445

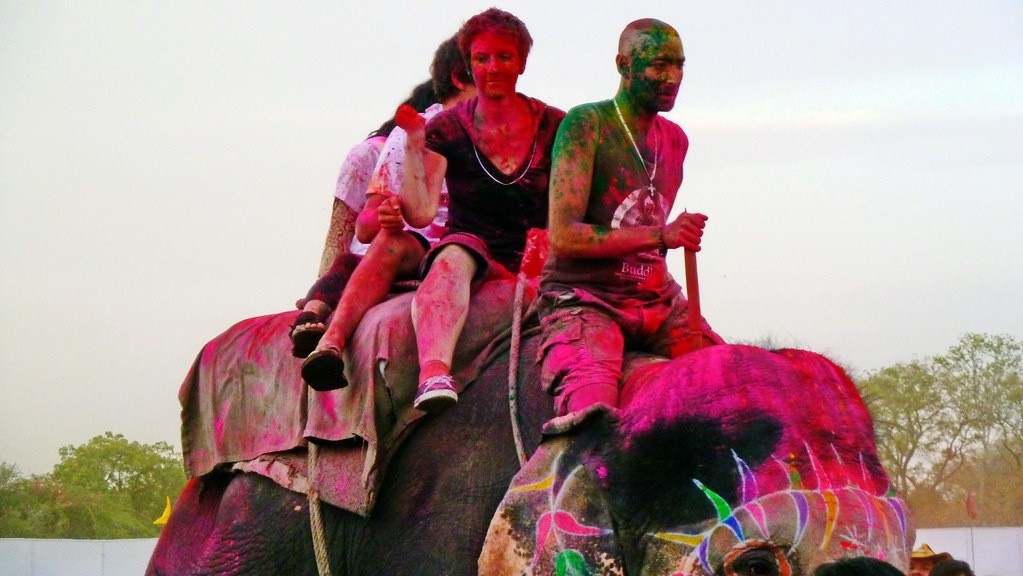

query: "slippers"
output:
287 311 327 359
300 345 348 392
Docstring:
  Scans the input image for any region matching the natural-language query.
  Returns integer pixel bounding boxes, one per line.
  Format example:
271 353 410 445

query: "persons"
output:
815 543 974 576
287 75 441 358
300 34 479 392
393 5 568 407
538 17 728 418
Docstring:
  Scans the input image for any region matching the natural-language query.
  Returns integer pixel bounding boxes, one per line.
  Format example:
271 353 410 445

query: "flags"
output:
967 492 977 519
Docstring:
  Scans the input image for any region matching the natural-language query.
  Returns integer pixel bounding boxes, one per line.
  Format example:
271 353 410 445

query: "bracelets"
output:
657 227 668 257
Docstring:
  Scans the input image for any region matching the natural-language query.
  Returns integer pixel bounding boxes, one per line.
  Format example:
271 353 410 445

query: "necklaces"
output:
470 91 537 185
613 96 658 196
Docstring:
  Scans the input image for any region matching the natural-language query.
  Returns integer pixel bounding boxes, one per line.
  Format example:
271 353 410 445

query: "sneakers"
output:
413 374 459 415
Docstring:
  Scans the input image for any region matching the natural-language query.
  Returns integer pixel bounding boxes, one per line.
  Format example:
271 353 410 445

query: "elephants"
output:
143 283 917 576
